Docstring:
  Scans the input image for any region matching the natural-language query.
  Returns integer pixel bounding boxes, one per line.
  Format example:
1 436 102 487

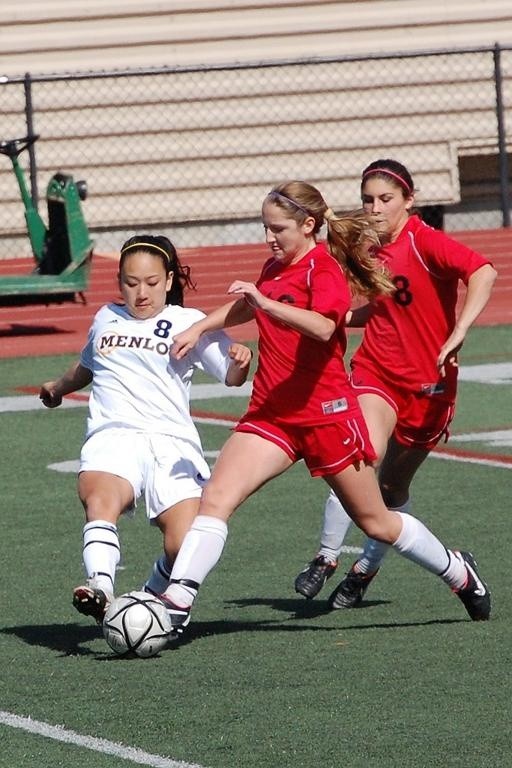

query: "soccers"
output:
102 591 174 660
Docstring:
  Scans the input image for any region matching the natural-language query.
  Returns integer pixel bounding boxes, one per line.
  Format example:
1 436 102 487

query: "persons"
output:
139 178 490 639
38 234 254 625
291 157 499 613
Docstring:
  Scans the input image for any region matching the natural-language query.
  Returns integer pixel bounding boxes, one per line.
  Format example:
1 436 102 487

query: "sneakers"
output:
327 560 380 609
294 554 338 601
451 550 491 621
72 585 111 625
156 594 191 638
